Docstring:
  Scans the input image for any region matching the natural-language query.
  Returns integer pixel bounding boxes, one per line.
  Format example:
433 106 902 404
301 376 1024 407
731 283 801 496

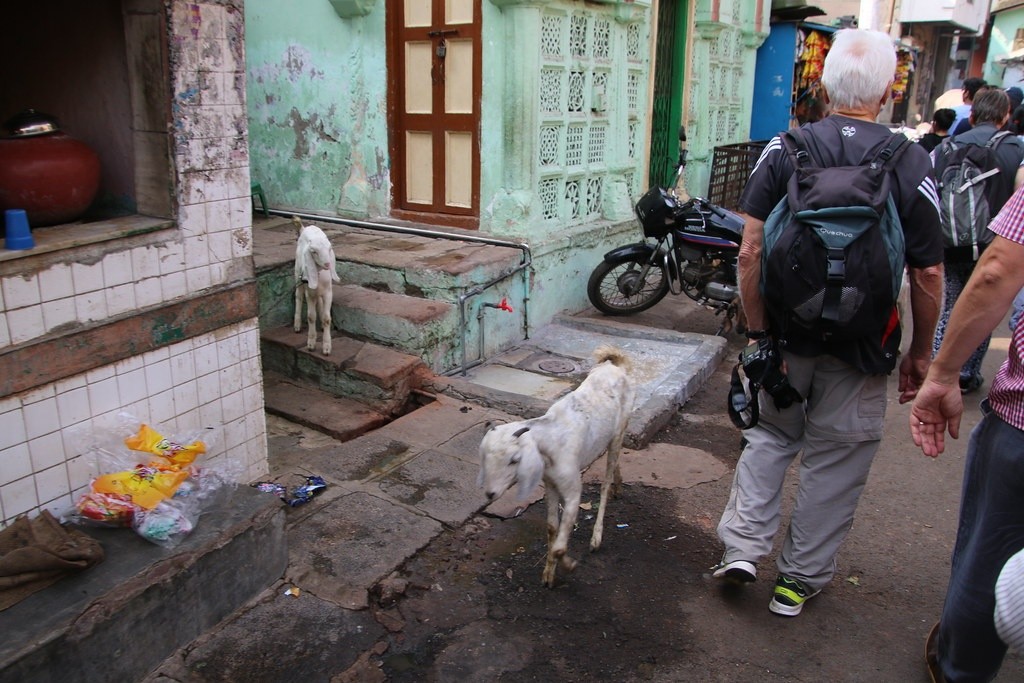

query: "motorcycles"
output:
588 126 746 336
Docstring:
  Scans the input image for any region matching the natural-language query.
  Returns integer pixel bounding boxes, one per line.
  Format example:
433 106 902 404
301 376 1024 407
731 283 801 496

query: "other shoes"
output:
924 621 942 683
959 374 984 393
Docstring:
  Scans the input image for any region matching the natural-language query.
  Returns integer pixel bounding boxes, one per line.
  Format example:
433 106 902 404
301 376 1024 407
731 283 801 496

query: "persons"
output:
909 182 1024 683
915 73 1024 396
706 30 945 616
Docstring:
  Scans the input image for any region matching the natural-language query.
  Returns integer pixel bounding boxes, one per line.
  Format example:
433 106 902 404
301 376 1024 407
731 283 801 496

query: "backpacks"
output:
934 130 1015 261
760 128 912 376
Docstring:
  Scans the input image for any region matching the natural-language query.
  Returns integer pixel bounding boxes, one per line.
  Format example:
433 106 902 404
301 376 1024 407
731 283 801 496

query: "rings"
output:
920 421 925 425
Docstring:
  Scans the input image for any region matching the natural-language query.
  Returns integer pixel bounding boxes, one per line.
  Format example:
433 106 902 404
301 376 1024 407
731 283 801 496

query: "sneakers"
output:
708 559 758 584
769 574 822 616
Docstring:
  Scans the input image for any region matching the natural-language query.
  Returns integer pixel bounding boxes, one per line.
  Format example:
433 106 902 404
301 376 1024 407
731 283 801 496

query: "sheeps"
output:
292 215 340 355
475 343 636 590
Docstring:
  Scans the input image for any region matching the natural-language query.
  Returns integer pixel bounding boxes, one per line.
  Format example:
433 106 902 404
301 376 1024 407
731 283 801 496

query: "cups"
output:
4 209 33 250
